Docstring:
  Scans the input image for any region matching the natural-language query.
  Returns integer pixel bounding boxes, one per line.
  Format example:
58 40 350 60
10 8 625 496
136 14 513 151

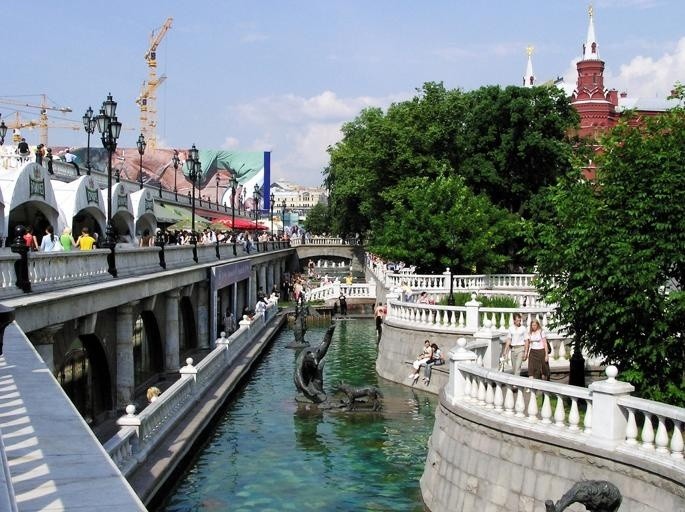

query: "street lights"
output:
94 92 123 250
444 232 458 316
135 134 146 184
82 105 97 166
171 145 288 246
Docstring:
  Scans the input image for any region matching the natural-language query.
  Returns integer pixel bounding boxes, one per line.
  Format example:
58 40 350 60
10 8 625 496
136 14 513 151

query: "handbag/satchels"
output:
546 340 553 355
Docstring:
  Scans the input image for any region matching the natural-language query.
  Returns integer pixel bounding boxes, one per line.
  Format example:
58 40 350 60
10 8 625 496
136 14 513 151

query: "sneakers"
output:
408 373 419 380
422 377 430 382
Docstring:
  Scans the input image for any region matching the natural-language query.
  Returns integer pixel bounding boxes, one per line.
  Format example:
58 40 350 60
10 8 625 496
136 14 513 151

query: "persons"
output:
14 137 82 177
146 383 162 403
423 343 445 383
503 312 527 390
415 292 430 316
522 320 550 393
409 340 432 381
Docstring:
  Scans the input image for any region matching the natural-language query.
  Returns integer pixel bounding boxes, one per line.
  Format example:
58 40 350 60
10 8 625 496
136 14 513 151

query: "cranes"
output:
134 73 166 151
0 94 79 148
144 17 174 153
